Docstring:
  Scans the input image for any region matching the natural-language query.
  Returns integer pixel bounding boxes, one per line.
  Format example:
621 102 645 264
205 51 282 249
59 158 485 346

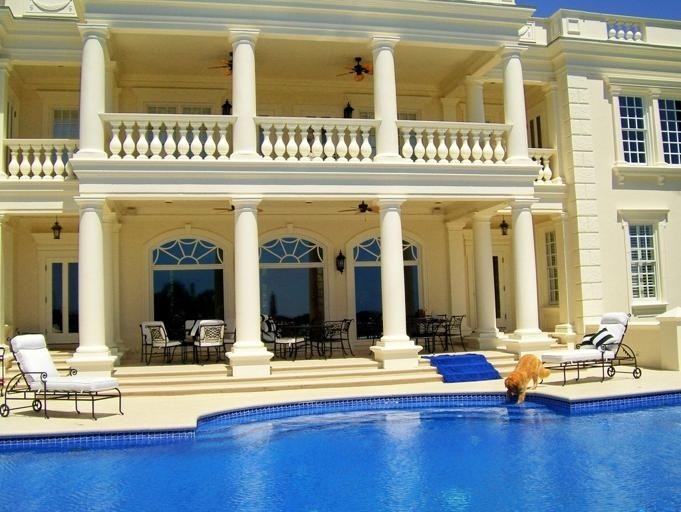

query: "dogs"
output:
503 353 552 405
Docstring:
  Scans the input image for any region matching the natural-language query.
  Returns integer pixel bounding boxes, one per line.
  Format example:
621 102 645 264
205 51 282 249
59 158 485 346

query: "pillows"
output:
580 334 596 348
590 328 616 350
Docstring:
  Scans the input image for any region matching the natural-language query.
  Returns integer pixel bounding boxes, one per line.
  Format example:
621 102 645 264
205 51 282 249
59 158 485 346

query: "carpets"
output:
431 354 504 383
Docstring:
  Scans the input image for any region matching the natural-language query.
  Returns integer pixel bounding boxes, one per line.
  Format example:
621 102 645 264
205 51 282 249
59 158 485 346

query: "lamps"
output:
222 101 232 115
344 102 353 118
51 216 62 239
500 216 508 235
336 250 345 274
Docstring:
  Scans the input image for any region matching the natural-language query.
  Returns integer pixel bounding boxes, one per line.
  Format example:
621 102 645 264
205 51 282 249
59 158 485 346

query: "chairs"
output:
140 320 236 365
370 310 467 355
0 334 124 420
261 314 355 361
539 313 641 387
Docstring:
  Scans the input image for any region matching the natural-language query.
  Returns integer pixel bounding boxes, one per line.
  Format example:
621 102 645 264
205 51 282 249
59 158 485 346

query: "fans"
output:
337 200 379 214
336 57 374 76
208 52 232 69
